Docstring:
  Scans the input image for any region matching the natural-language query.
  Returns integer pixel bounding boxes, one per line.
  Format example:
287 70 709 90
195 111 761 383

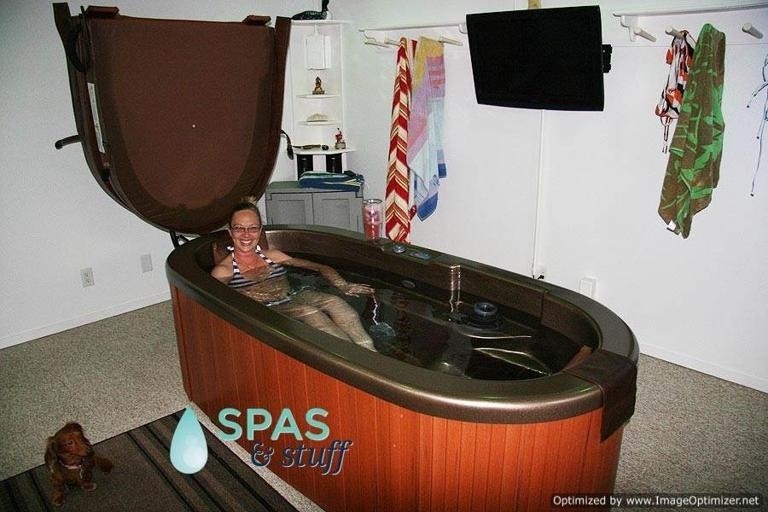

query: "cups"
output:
362 199 384 244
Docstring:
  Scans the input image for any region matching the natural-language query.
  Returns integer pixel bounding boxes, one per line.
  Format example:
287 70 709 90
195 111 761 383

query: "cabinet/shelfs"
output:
264 179 365 235
288 19 353 181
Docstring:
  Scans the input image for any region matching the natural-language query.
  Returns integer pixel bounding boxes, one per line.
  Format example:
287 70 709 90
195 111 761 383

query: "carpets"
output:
0 406 301 512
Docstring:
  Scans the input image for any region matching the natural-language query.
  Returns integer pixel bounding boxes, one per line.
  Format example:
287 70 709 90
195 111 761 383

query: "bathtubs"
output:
165 223 640 512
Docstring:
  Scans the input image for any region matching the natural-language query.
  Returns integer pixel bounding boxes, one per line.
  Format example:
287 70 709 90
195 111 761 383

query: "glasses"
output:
229 224 261 233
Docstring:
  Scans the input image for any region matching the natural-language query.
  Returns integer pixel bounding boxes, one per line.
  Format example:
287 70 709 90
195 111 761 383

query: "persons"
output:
212 201 377 354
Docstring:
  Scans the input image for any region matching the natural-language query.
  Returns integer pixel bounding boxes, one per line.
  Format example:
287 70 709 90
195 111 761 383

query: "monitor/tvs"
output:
466 5 612 111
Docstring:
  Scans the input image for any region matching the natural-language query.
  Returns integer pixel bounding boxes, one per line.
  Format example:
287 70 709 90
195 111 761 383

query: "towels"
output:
383 35 416 245
404 36 449 221
657 21 727 240
654 29 696 156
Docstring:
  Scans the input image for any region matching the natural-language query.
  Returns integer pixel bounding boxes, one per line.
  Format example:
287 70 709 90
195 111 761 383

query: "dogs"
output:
44 421 114 508
